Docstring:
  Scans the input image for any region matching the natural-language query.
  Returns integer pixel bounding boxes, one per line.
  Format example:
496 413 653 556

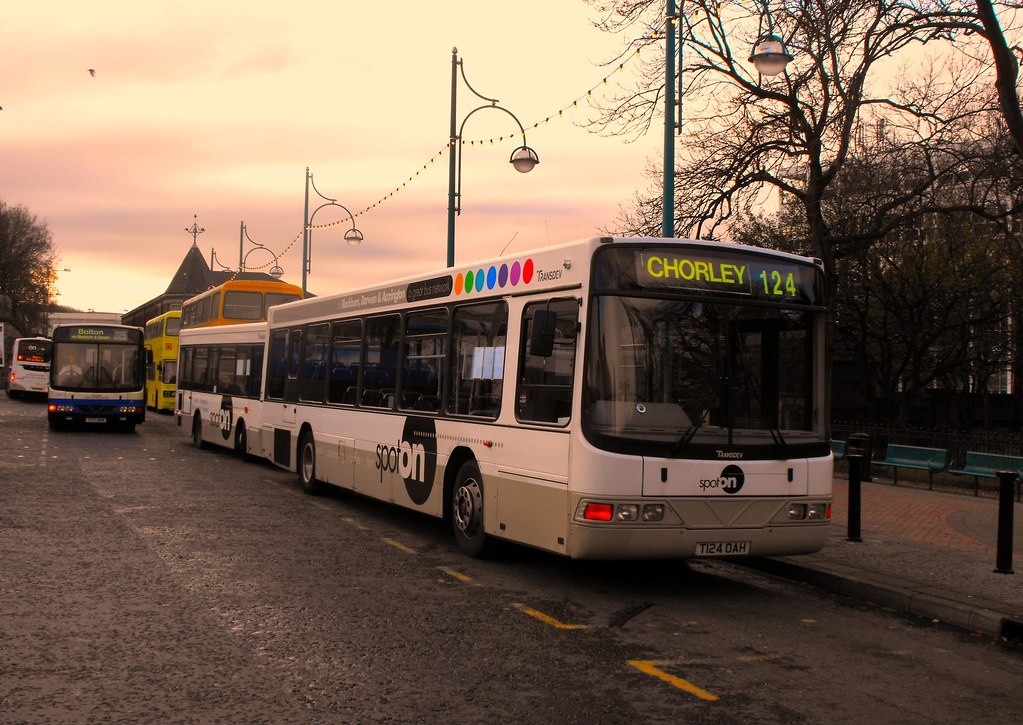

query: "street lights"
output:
663 0 794 237
302 166 363 292
237 220 284 280
447 45 540 267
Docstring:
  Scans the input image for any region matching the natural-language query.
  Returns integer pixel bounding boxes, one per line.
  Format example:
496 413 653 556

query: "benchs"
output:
831 439 846 461
948 451 1023 502
870 443 947 490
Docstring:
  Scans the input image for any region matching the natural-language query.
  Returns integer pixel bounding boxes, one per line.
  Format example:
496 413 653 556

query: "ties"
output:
70 366 73 375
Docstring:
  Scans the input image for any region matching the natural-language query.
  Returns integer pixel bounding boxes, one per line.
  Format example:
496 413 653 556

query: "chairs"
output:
274 357 439 410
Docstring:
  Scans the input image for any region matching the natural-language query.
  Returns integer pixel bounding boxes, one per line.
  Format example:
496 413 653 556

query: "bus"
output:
145 311 182 414
260 238 834 561
174 322 272 465
6 336 54 399
43 323 153 430
177 278 305 329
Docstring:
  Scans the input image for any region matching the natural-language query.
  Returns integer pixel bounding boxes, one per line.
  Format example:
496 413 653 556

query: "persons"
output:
59 355 83 375
